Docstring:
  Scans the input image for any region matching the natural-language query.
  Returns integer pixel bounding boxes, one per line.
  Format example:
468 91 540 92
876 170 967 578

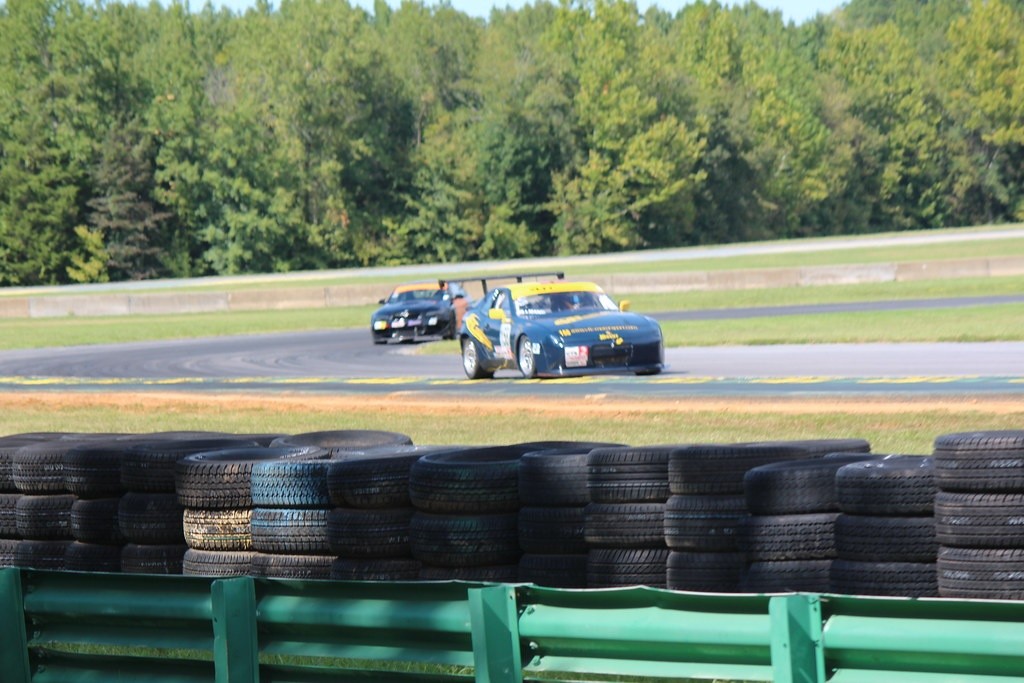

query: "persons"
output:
557 294 586 312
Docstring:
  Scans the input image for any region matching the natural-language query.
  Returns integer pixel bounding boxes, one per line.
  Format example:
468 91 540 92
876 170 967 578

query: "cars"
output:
459 281 665 379
370 282 475 343
